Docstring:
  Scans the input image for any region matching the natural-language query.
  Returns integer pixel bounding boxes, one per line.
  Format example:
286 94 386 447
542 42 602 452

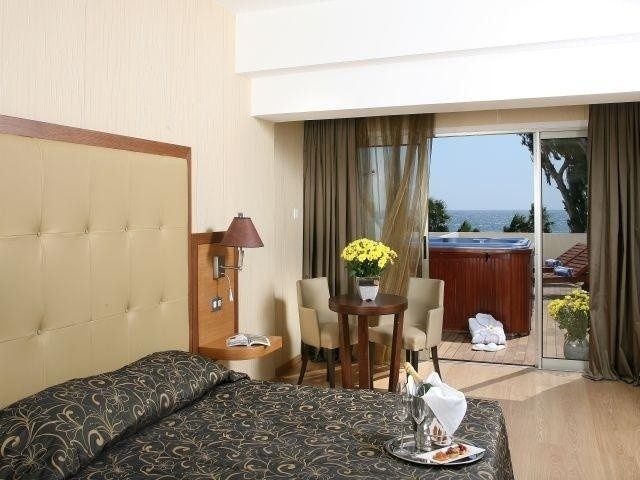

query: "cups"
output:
414 397 453 452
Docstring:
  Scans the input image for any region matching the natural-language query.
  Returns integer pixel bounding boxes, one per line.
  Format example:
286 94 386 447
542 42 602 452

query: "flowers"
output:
546 288 590 345
338 237 399 277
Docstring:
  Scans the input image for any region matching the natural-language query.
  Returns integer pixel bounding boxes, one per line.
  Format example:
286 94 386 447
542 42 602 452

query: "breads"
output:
446 447 459 458
455 444 467 454
433 452 450 461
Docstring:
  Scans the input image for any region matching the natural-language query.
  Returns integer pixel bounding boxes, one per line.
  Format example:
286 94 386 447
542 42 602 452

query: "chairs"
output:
542 241 587 270
295 276 358 388
368 276 445 383
542 248 590 283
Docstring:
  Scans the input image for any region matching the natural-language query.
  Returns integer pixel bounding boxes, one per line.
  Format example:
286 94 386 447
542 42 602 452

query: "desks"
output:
198 331 284 361
327 294 409 393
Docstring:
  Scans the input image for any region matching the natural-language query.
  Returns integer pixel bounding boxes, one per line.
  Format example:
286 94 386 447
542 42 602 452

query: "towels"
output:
552 265 575 278
544 259 563 267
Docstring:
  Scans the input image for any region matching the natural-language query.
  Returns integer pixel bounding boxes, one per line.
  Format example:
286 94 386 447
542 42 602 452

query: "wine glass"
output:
396 381 410 455
411 386 425 460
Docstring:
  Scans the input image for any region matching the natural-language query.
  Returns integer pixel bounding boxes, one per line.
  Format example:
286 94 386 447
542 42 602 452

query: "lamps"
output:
213 211 265 304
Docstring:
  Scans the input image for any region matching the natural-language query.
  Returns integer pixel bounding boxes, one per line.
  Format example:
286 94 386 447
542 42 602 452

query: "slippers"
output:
472 343 505 352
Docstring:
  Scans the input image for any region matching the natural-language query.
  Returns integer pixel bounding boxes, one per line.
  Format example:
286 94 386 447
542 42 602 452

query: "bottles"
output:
402 361 432 395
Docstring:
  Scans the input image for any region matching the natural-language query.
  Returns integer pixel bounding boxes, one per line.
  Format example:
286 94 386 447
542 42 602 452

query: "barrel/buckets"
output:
409 387 453 453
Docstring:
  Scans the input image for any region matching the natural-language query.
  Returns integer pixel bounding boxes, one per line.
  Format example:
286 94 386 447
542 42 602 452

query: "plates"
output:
415 444 486 464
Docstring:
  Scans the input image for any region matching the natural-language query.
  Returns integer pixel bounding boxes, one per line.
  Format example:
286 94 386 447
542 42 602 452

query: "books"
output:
226 334 271 348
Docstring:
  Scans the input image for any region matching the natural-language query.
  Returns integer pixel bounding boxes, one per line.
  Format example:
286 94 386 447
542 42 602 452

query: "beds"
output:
1 113 520 479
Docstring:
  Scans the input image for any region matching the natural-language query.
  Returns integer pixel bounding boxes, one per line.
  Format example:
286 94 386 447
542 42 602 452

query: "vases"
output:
562 333 590 361
357 278 381 302
